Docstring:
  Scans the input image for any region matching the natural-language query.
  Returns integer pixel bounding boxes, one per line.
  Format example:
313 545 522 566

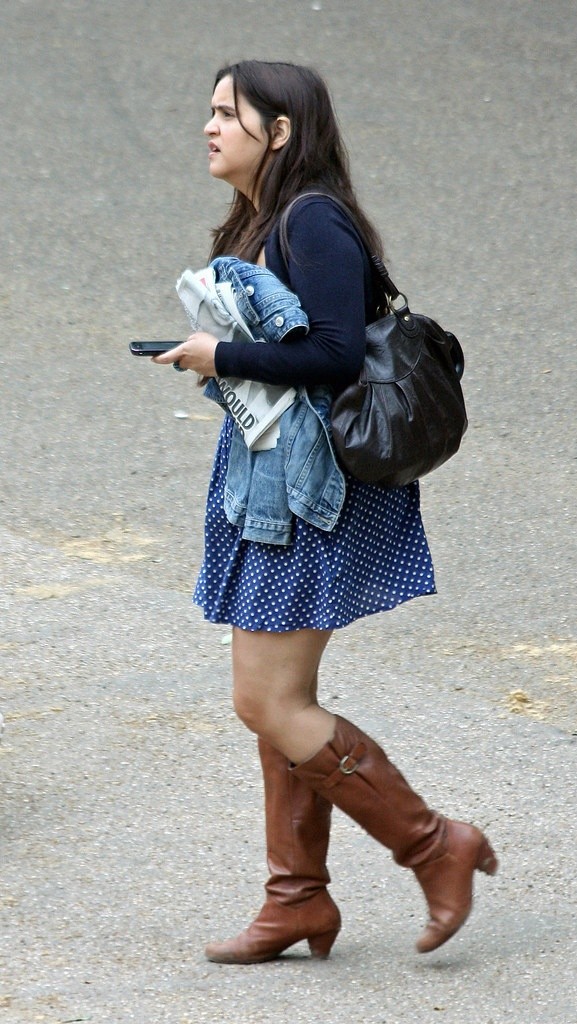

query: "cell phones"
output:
129 341 187 356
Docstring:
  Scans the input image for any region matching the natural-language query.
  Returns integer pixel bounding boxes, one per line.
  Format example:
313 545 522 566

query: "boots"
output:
207 737 340 964
289 714 499 953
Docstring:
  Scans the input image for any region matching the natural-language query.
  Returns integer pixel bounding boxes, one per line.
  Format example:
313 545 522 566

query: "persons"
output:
152 61 498 965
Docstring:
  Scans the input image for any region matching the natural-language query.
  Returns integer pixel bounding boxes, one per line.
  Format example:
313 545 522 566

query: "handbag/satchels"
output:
280 193 468 485
